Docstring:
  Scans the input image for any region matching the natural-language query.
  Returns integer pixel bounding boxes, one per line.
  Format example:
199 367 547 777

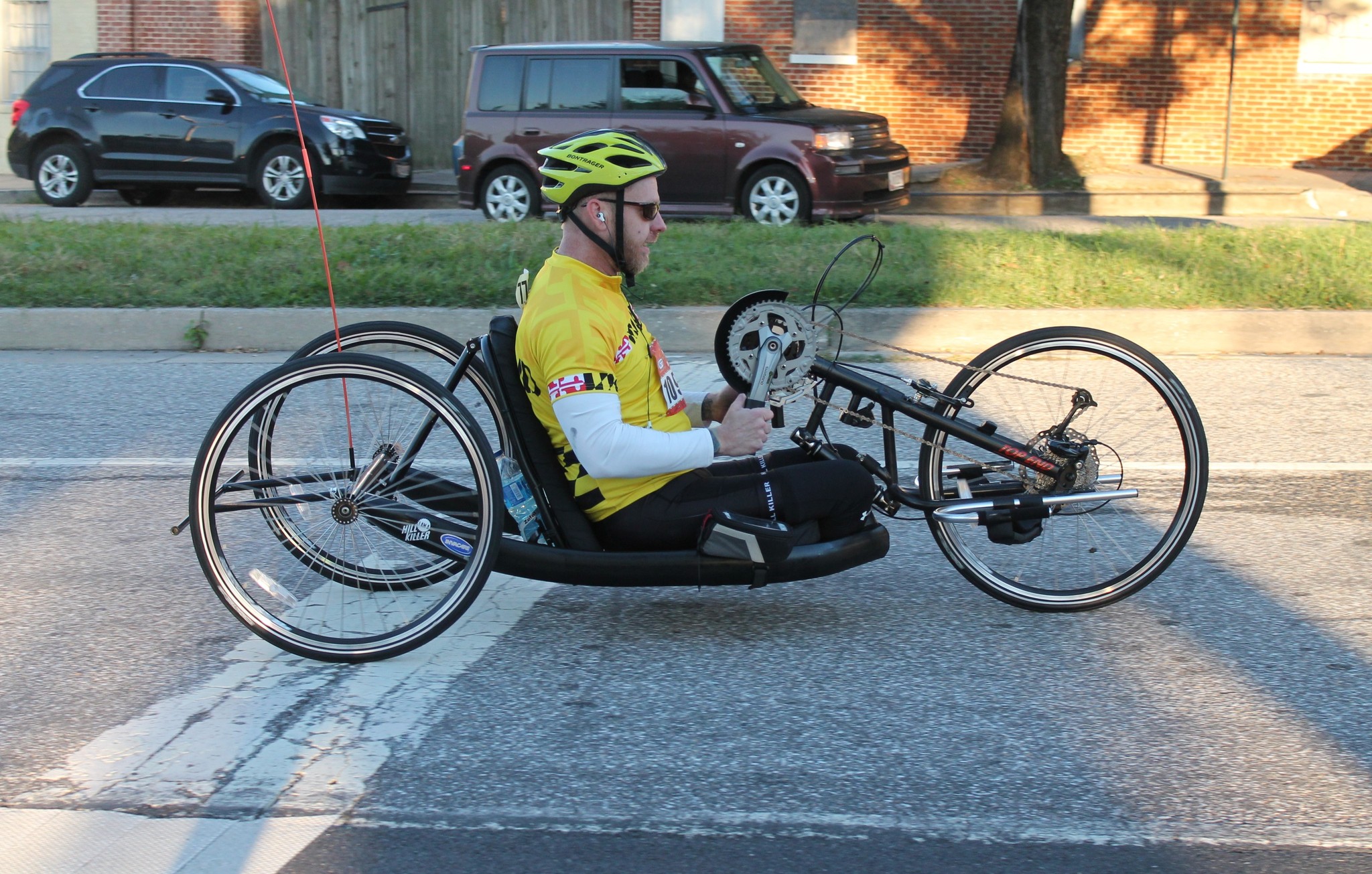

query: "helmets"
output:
537 128 666 222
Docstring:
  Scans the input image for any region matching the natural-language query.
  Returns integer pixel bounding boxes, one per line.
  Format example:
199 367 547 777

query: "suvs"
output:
8 50 417 209
454 42 914 229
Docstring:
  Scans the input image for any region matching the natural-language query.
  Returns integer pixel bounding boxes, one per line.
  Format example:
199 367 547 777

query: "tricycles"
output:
170 233 1213 663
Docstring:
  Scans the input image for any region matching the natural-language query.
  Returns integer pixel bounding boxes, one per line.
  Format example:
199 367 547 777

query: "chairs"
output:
625 69 664 111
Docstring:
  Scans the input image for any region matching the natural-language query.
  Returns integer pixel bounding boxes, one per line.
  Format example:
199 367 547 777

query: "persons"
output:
516 126 882 550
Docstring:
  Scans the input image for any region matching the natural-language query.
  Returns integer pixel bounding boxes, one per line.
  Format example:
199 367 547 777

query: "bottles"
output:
494 449 546 545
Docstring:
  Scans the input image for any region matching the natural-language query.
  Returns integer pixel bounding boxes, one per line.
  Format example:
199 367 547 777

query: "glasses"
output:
580 198 660 221
